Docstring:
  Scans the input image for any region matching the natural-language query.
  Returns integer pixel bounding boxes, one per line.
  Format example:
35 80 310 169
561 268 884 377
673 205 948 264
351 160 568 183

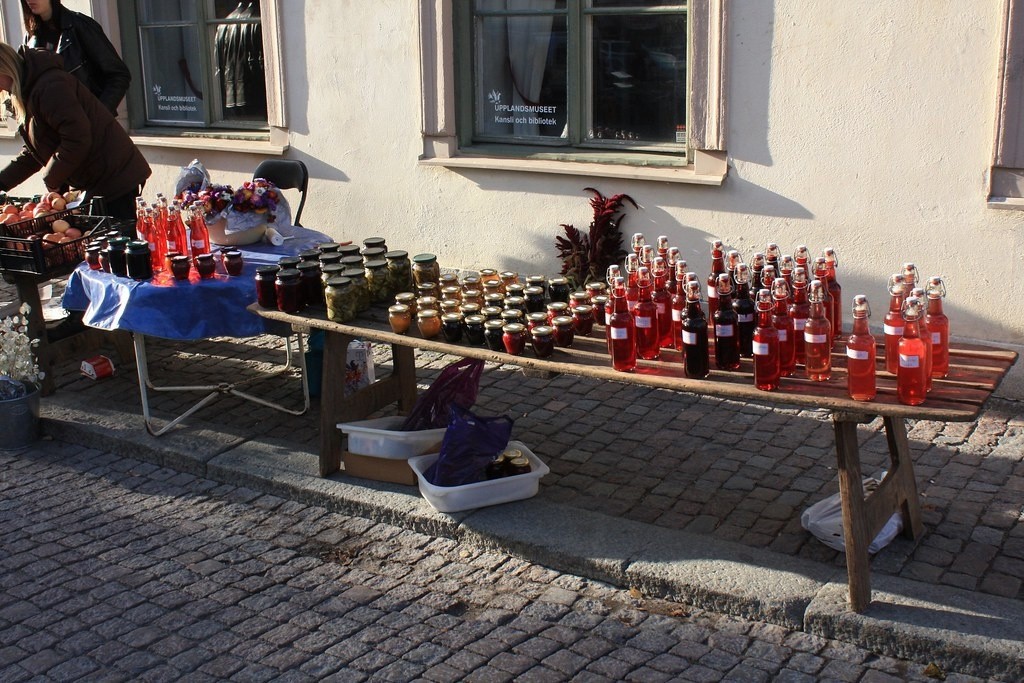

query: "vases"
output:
0 381 42 451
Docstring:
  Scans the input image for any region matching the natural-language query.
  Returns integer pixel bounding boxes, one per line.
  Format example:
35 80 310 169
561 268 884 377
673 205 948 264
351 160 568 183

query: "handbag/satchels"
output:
801 471 905 555
423 407 515 487
399 357 485 431
344 341 383 422
305 329 324 396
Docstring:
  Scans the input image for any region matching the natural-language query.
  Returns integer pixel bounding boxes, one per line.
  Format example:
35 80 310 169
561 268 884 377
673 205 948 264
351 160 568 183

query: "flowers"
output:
0 301 45 401
555 185 638 292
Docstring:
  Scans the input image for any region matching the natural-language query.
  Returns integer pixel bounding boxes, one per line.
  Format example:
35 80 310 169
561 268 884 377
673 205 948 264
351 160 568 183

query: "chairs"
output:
252 158 308 228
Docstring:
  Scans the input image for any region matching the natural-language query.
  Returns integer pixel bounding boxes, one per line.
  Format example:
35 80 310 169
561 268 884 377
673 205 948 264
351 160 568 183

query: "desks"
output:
245 286 1019 615
62 228 336 436
0 266 136 394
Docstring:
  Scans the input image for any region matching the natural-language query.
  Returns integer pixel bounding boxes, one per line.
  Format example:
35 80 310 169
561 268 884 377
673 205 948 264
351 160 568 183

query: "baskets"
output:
0 196 95 237
0 214 113 276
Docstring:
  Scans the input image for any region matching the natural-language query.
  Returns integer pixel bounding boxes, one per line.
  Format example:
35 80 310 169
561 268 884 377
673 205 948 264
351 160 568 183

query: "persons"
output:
212 1 265 108
19 0 132 118
0 42 152 325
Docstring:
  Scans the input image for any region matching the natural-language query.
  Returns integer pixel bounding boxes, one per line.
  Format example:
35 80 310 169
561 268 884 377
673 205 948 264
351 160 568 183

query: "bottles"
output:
883 263 949 405
389 269 610 359
265 228 285 245
846 294 876 401
708 241 843 391
605 233 710 379
488 448 532 480
84 230 156 279
255 237 440 321
164 244 243 281
135 192 211 271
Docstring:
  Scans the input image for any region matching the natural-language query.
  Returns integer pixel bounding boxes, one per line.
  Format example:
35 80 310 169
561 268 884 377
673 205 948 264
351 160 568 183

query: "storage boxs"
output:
409 440 552 512
0 193 115 274
338 416 448 460
344 441 445 485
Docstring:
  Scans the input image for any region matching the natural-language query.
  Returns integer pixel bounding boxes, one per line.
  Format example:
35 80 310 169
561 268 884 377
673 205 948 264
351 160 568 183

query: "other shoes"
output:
47 313 92 344
